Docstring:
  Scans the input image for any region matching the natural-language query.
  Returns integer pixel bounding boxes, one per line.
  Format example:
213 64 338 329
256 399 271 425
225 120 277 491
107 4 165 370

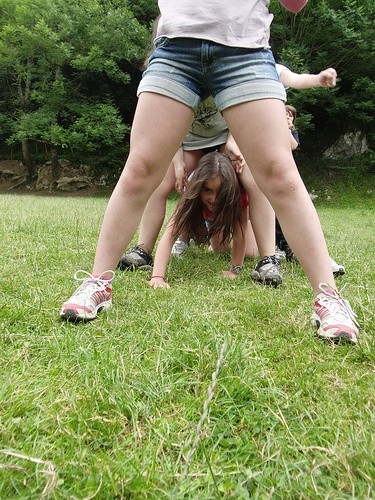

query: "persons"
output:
117 14 337 290
60 0 360 346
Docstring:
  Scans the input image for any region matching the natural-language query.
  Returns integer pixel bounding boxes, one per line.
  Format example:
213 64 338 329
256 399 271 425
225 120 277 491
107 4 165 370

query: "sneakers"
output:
313 290 360 344
59 275 113 321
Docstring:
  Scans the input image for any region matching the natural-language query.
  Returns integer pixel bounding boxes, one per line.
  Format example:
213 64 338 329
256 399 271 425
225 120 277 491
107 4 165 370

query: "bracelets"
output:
151 276 164 279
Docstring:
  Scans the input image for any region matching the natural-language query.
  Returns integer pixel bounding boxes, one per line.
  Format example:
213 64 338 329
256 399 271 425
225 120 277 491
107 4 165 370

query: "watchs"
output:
229 265 244 274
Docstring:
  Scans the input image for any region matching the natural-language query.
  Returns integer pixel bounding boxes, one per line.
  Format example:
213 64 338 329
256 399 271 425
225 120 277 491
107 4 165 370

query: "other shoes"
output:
117 245 153 271
329 255 345 275
171 238 190 256
250 255 283 287
